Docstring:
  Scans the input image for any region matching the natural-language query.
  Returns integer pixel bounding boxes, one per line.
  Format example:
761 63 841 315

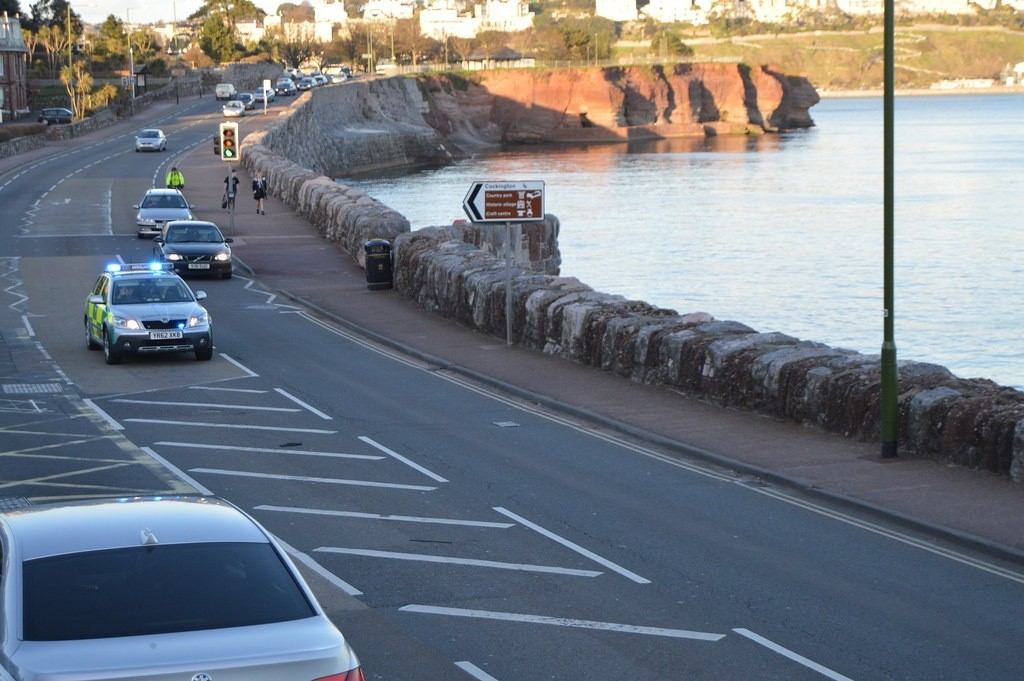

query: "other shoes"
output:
257 209 259 214
262 211 265 215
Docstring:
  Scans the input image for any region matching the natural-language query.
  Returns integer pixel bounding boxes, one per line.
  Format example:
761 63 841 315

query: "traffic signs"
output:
462 179 544 223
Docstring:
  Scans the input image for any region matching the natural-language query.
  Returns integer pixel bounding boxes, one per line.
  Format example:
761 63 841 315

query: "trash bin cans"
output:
364 238 394 292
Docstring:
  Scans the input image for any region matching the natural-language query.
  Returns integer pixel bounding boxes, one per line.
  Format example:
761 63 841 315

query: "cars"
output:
297 67 352 91
135 129 168 152
235 92 254 110
0 494 363 681
153 221 234 280
275 78 296 95
253 87 275 102
222 101 245 117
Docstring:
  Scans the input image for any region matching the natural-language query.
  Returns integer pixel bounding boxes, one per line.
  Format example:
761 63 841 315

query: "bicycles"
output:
170 185 184 189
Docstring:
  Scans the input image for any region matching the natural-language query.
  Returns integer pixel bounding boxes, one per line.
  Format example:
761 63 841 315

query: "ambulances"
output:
84 263 213 365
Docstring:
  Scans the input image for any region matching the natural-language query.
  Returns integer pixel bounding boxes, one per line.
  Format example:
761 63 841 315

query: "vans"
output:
215 83 237 101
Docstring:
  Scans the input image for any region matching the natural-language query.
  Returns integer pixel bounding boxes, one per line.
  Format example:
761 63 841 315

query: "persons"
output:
251 172 267 215
114 286 140 303
223 169 240 213
166 167 185 191
150 286 178 302
198 233 212 242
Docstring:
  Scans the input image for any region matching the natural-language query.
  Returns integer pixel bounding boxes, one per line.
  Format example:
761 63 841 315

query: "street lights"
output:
67 1 98 124
126 5 151 95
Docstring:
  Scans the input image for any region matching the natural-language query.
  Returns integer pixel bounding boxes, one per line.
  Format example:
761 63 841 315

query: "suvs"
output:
38 107 76 126
132 186 195 240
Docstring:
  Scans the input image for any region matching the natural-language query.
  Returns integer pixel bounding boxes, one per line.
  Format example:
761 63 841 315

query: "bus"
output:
376 65 396 75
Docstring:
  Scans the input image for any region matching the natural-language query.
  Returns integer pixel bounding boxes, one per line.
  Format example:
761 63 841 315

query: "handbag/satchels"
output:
222 193 227 208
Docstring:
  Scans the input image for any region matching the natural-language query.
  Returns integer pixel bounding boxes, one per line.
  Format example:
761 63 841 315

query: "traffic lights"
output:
223 126 236 159
213 135 220 155
